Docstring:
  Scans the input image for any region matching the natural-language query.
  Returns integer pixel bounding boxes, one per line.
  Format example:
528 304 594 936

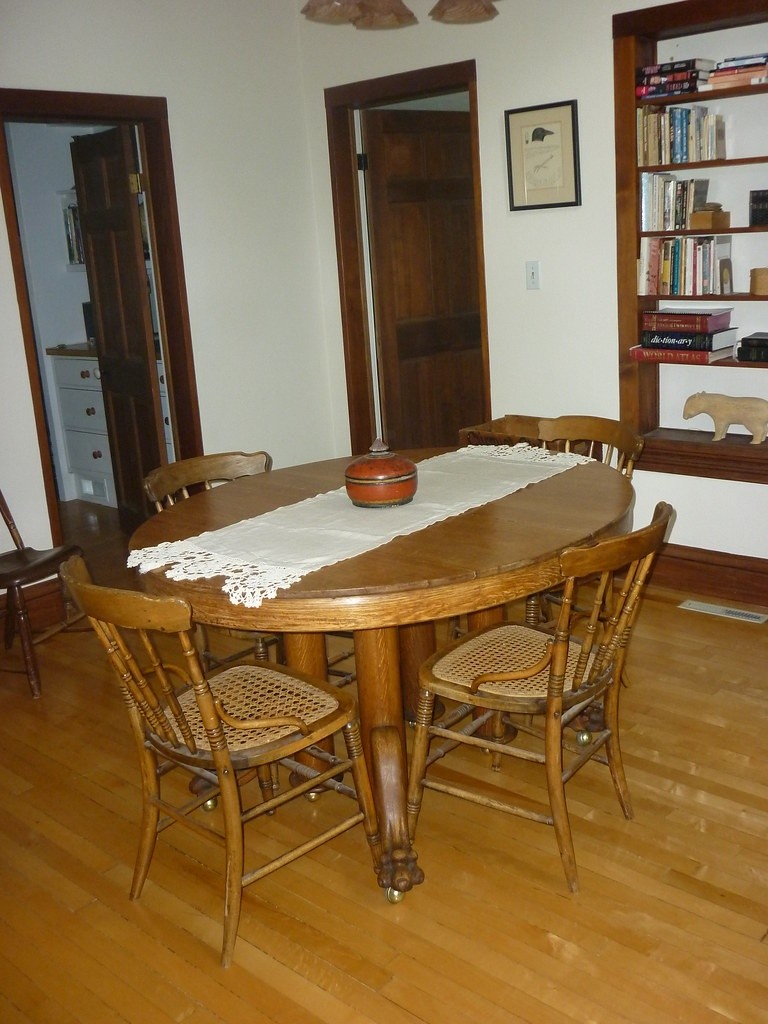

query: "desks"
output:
129 447 636 799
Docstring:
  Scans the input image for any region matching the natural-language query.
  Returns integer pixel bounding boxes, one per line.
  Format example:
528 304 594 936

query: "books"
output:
627 308 738 365
737 331 768 363
697 53 768 92
640 172 709 231
635 58 715 99
749 190 768 227
638 236 732 294
637 104 726 166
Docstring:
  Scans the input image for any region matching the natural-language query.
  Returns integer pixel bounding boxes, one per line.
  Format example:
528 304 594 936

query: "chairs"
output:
57 553 384 971
447 415 644 727
0 485 108 699
143 451 358 788
408 500 674 884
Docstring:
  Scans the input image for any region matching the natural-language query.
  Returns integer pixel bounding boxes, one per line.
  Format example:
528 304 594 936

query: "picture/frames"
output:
504 99 582 211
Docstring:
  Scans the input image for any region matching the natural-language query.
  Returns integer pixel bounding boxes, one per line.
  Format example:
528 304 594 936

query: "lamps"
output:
301 0 499 30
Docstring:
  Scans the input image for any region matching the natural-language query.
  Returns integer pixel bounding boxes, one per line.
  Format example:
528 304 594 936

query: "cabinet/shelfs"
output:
44 353 174 509
612 1 768 484
